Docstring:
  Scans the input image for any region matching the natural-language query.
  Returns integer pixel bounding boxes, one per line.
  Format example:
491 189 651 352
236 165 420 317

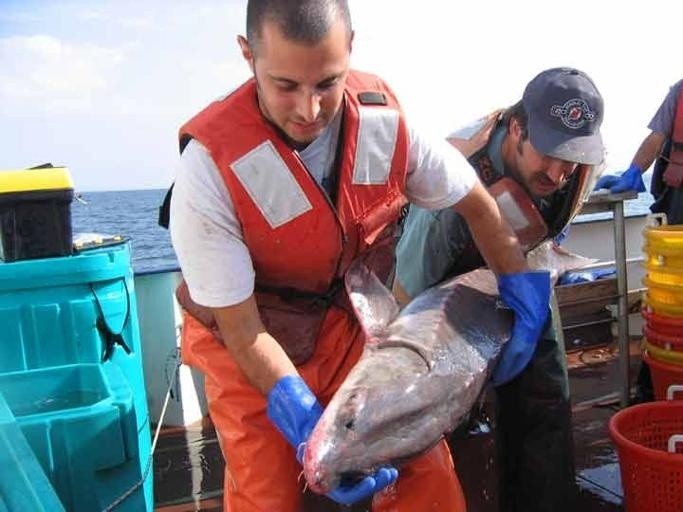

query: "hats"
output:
522 66 605 165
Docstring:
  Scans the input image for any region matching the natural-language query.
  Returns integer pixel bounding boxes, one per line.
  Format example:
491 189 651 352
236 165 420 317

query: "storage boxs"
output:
0 162 89 263
0 237 156 512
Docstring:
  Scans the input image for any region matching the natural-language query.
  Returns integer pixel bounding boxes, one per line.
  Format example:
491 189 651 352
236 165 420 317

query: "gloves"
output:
491 269 553 389
559 263 617 287
554 223 571 248
594 164 647 194
264 374 401 506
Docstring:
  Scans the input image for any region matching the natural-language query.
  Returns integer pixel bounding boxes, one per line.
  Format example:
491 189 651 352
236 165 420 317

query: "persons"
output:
594 79 683 409
387 67 605 512
157 0 551 512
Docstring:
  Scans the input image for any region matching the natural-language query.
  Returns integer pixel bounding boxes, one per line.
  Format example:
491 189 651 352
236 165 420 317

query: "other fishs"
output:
296 237 598 496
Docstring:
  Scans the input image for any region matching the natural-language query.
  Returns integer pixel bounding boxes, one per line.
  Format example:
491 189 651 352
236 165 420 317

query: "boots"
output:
627 360 654 406
